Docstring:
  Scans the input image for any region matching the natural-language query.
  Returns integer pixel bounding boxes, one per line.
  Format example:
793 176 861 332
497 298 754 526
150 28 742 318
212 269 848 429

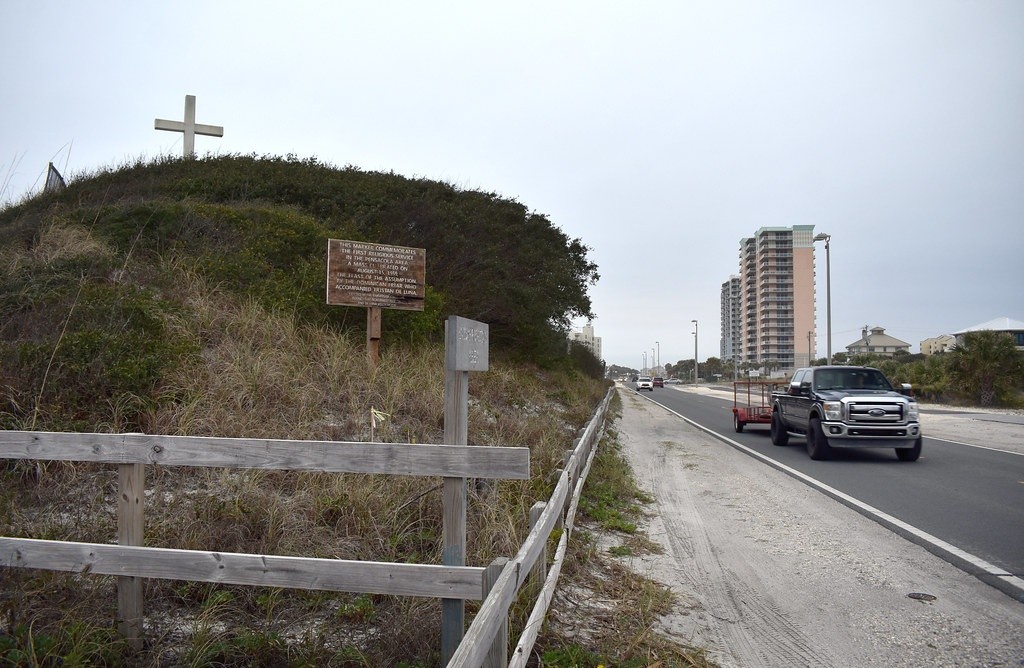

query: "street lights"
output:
656 342 659 373
692 320 697 388
813 233 832 366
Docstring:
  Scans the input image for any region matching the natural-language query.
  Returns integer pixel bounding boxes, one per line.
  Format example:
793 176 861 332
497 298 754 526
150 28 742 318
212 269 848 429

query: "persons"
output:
856 375 867 388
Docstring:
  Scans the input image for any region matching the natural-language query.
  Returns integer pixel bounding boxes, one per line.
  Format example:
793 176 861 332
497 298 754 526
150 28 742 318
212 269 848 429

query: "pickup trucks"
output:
771 365 922 463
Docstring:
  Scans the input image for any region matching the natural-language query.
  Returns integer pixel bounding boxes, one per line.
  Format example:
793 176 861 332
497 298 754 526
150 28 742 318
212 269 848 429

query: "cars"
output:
663 378 684 385
653 378 663 388
636 377 654 391
